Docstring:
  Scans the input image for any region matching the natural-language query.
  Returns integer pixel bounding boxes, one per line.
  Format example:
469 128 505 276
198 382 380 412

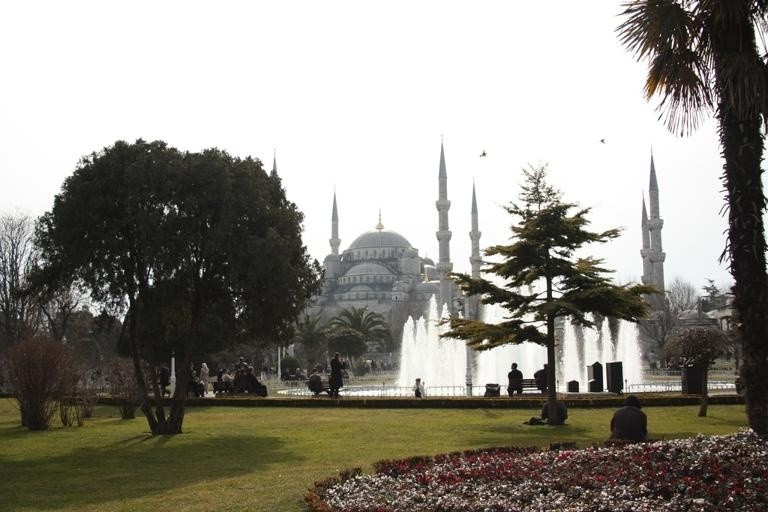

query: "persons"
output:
329 351 346 398
158 367 171 396
189 355 269 396
507 362 523 396
541 391 569 426
412 378 426 397
308 368 324 397
534 364 548 394
610 395 647 440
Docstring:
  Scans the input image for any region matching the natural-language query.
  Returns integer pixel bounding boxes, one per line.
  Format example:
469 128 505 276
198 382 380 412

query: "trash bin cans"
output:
569 381 579 392
485 384 500 397
587 362 623 392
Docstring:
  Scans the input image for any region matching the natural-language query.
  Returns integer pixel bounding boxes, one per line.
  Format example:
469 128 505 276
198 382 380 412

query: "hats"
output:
623 396 641 409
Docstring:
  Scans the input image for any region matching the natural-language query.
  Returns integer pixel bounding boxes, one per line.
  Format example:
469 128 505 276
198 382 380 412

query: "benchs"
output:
509 376 550 395
233 382 265 397
213 381 233 393
306 380 344 397
187 384 204 397
